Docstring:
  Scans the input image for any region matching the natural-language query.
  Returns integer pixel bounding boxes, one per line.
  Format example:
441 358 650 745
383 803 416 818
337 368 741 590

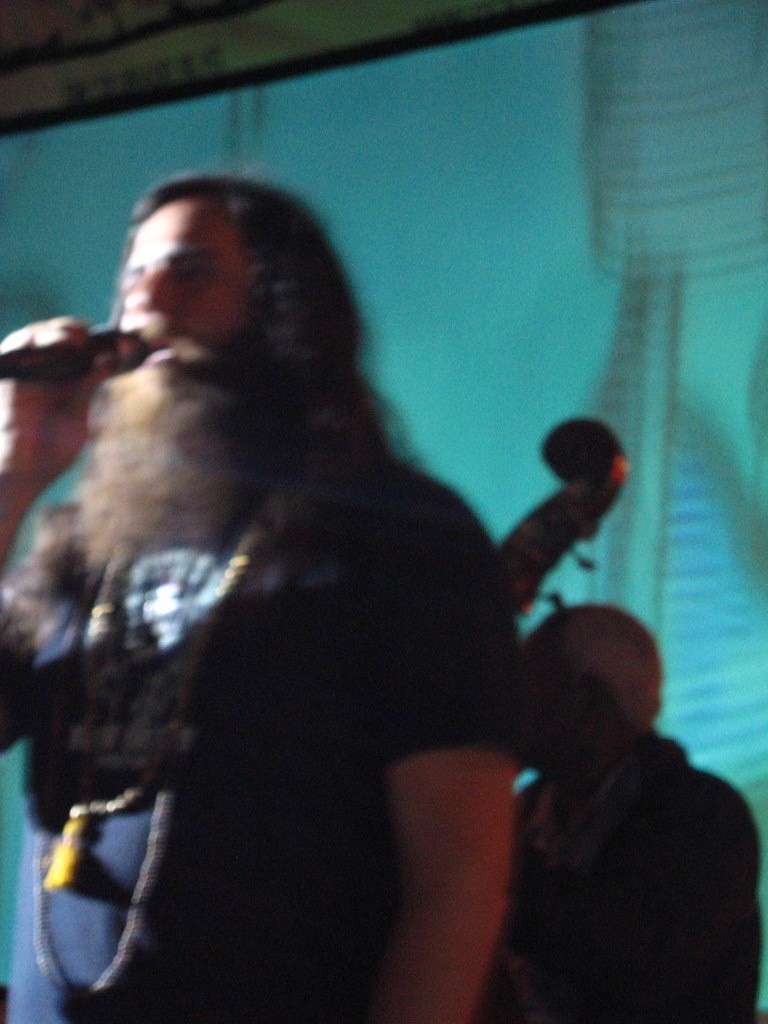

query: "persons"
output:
472 605 761 1024
0 171 520 1024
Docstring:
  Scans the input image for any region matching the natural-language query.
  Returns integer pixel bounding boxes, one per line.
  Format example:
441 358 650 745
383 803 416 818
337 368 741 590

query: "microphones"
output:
0 326 149 382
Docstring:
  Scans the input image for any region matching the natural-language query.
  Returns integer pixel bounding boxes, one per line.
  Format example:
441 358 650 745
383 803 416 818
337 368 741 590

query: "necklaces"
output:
32 498 267 998
